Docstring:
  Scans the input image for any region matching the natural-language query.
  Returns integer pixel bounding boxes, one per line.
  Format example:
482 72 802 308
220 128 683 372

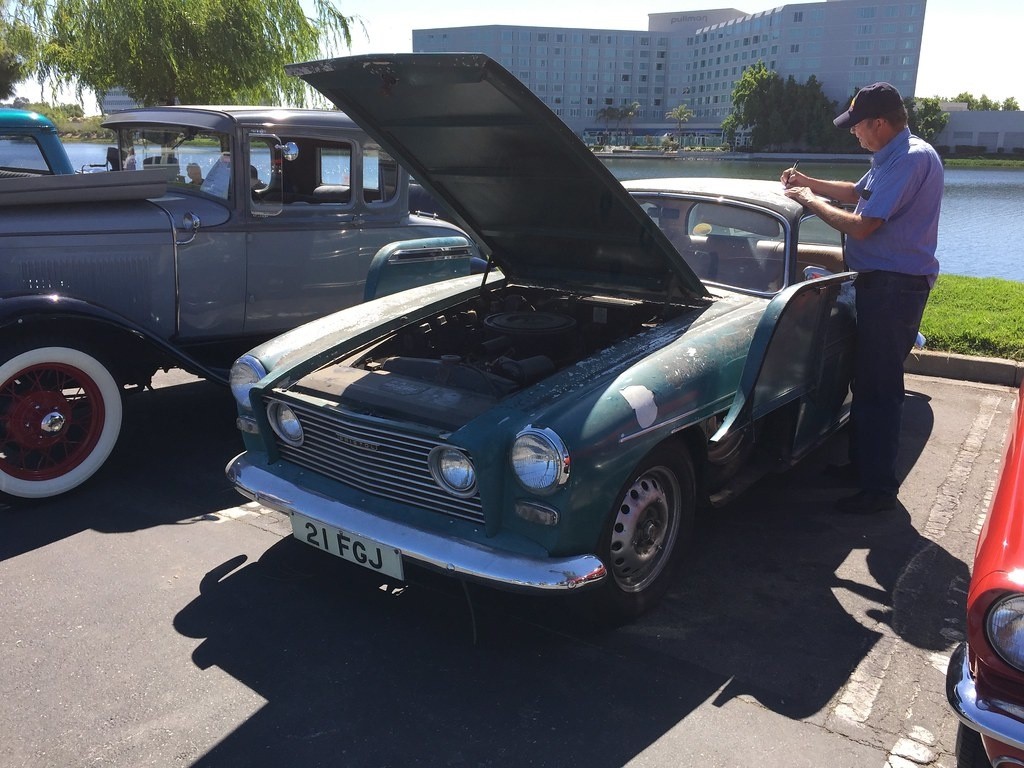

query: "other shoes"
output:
825 460 869 492
837 481 899 514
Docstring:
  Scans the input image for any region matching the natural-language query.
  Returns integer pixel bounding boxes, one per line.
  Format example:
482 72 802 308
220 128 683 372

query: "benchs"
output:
668 233 846 297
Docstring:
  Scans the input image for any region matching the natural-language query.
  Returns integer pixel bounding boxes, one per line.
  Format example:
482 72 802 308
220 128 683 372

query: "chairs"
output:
80 145 127 173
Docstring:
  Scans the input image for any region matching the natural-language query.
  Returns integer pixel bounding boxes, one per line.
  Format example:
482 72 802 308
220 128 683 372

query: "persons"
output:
251 164 267 189
122 146 137 170
186 162 205 185
781 82 944 514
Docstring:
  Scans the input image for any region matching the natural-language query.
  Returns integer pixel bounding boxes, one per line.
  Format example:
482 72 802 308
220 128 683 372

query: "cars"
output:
224 52 857 632
946 386 1024 768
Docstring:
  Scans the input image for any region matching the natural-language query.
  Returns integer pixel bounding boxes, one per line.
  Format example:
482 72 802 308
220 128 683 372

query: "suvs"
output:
0 108 75 178
0 105 496 511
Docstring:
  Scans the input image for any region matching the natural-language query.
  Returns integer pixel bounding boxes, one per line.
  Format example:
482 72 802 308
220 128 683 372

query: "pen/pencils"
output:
785 159 801 185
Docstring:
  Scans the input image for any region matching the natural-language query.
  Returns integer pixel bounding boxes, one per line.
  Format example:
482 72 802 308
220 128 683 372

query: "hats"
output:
833 82 904 131
